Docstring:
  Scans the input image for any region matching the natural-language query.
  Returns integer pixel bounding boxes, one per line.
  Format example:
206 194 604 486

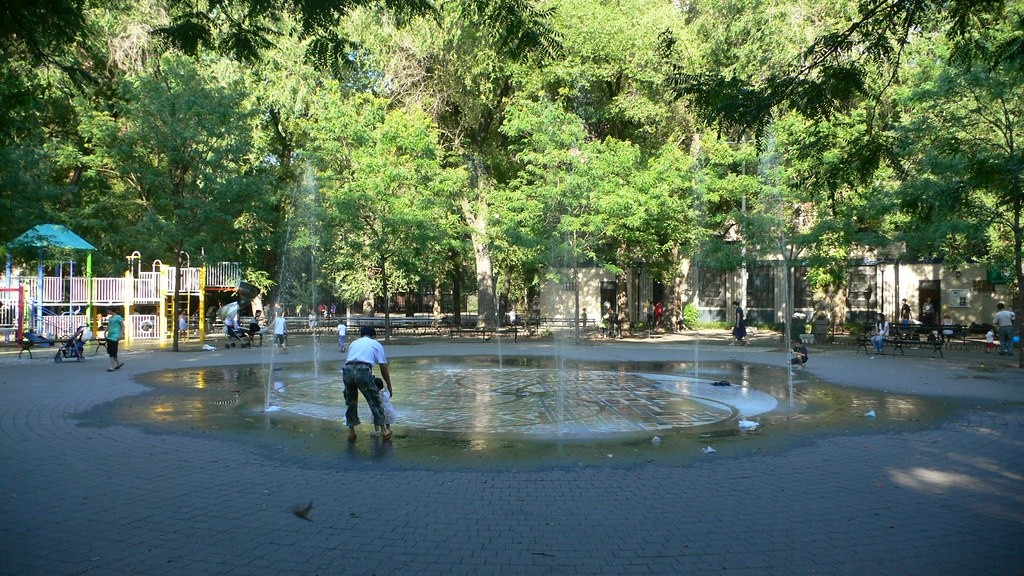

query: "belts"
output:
346 362 372 370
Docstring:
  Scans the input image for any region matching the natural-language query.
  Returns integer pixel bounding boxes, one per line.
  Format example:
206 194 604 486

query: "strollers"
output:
222 315 249 349
54 329 87 363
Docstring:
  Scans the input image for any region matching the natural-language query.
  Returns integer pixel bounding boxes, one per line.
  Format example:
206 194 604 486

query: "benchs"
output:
537 317 595 334
15 338 108 359
857 322 968 358
450 327 519 343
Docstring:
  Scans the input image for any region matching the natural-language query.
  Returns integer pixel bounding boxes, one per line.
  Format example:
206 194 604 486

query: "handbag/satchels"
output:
599 317 606 328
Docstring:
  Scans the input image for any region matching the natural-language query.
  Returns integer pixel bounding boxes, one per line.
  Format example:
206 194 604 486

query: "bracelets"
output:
387 384 391 388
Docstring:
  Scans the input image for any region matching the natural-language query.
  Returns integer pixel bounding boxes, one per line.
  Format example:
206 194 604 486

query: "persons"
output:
985 327 995 353
580 298 689 339
177 309 188 341
787 338 808 369
192 301 289 353
370 378 393 437
728 301 751 346
871 313 890 353
295 303 346 352
898 297 936 335
993 303 1016 356
104 306 125 372
105 308 112 321
342 325 393 442
509 309 516 324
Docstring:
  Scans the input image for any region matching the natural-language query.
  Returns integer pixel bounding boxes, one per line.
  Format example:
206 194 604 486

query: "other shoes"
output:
372 431 380 436
340 348 345 352
348 435 356 440
1009 352 1013 356
729 342 736 346
283 347 288 354
115 363 124 369
107 368 115 371
798 364 805 369
1001 352 1006 355
384 433 391 440
744 343 751 346
390 430 393 435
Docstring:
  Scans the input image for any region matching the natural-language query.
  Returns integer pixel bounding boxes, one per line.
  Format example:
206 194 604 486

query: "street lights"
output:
879 263 887 315
636 267 643 321
